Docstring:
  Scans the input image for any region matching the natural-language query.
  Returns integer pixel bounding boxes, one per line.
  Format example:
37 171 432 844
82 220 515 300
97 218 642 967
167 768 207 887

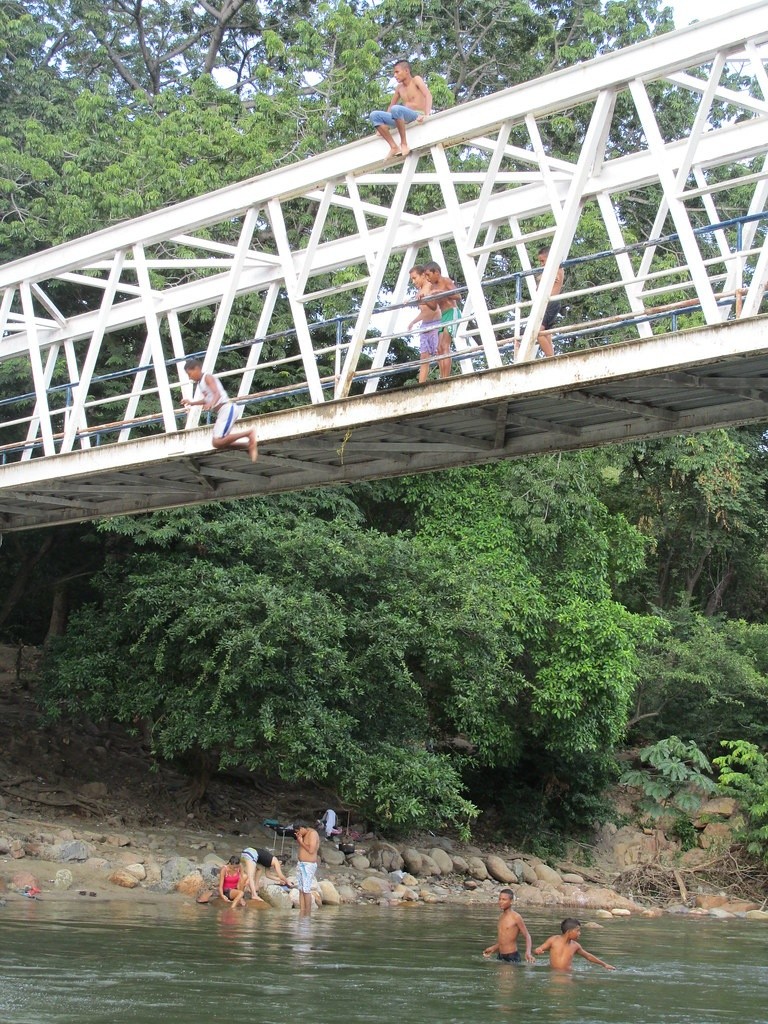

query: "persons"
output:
320 807 338 843
482 888 536 964
217 856 248 909
293 818 320 919
417 262 462 380
536 246 565 360
532 918 617 973
180 360 258 462
370 60 432 165
242 846 294 902
407 264 442 385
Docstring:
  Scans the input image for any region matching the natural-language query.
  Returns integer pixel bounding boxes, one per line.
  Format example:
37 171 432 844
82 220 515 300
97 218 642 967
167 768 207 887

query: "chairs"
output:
259 819 297 855
